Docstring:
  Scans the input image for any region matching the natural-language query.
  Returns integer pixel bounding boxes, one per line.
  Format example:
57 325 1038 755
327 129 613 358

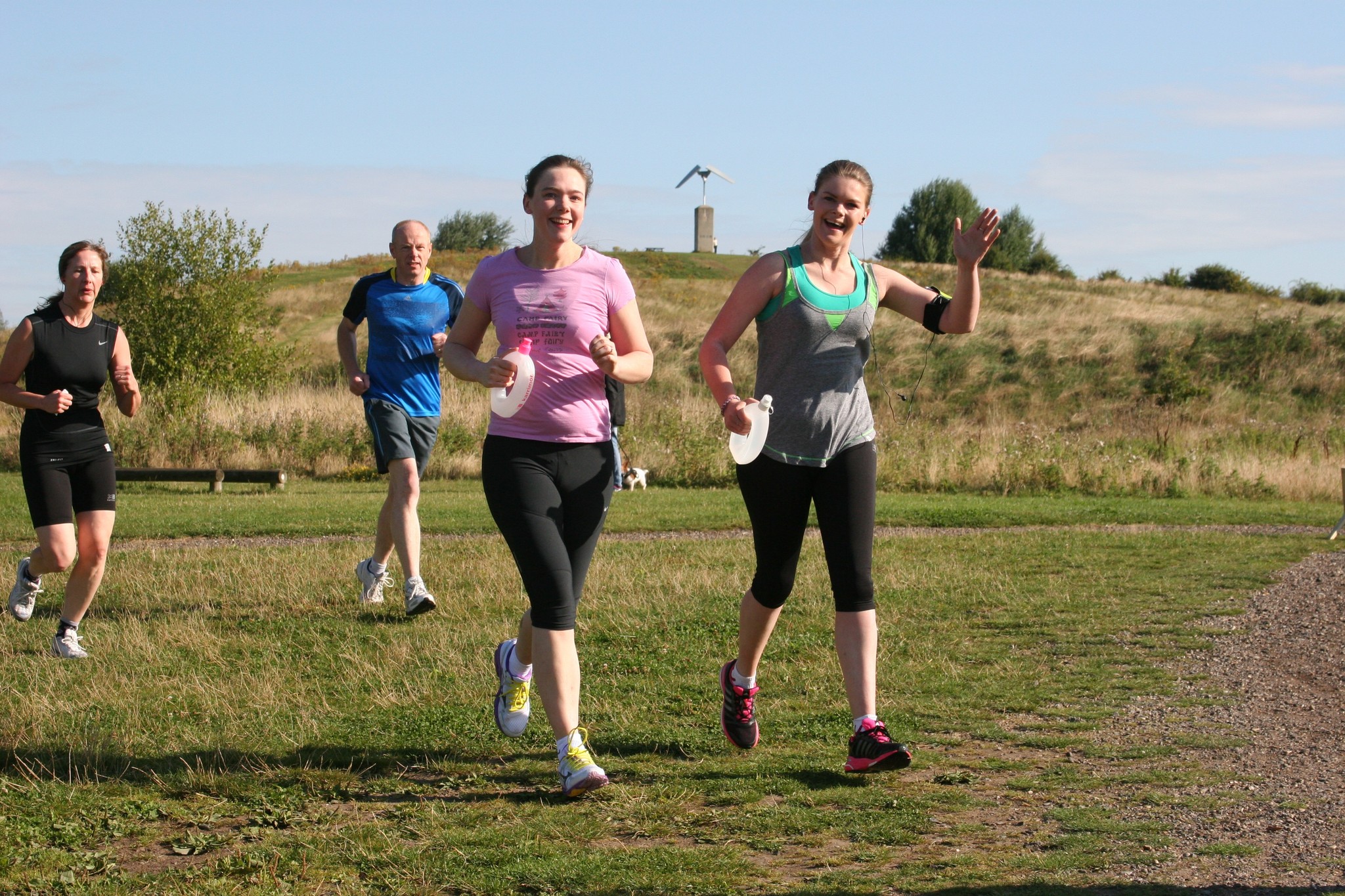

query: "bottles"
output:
491 338 535 418
728 394 774 465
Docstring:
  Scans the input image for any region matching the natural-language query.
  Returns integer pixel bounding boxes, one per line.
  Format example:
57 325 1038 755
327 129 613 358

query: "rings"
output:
737 419 740 425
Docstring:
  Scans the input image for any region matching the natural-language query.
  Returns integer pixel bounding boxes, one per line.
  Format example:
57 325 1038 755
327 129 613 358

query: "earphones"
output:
862 216 865 223
898 393 906 401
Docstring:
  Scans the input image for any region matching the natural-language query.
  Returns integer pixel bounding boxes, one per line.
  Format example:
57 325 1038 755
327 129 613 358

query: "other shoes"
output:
614 485 622 491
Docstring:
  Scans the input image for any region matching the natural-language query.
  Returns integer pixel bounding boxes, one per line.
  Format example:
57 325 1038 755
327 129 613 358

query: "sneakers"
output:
494 638 532 737
356 557 395 603
403 576 436 615
844 717 912 773
719 658 760 750
51 629 87 659
557 723 608 796
9 557 43 623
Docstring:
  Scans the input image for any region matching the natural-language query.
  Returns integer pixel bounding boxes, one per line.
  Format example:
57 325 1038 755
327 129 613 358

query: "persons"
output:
713 237 717 254
0 241 141 658
698 159 1001 774
603 372 625 491
336 219 464 615
443 153 654 794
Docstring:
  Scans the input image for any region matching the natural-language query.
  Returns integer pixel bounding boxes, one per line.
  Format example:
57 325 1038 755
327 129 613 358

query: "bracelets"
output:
721 395 741 416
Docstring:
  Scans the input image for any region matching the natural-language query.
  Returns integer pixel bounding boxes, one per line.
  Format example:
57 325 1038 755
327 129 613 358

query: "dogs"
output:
620 457 650 492
